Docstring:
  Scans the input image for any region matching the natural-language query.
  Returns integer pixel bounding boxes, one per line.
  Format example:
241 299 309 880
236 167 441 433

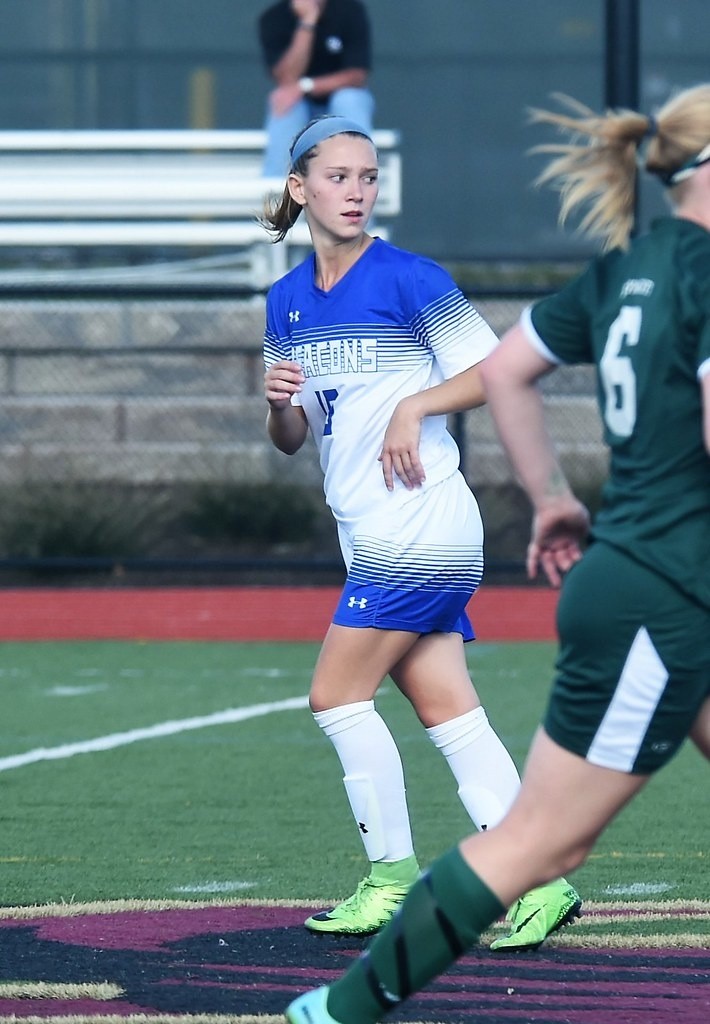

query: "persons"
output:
254 116 583 949
257 0 376 178
286 83 709 1023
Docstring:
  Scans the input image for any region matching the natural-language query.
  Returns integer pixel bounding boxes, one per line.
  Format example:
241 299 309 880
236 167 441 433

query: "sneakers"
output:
489 875 584 952
284 985 341 1024
303 876 422 940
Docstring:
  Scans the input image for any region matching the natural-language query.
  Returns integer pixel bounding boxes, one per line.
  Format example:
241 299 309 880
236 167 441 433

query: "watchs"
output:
299 77 314 94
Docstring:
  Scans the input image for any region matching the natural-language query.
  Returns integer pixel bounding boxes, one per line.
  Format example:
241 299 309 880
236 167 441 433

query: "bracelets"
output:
296 21 317 29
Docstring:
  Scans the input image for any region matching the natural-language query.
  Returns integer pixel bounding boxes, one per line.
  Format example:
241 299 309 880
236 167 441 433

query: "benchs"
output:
1 125 402 290
